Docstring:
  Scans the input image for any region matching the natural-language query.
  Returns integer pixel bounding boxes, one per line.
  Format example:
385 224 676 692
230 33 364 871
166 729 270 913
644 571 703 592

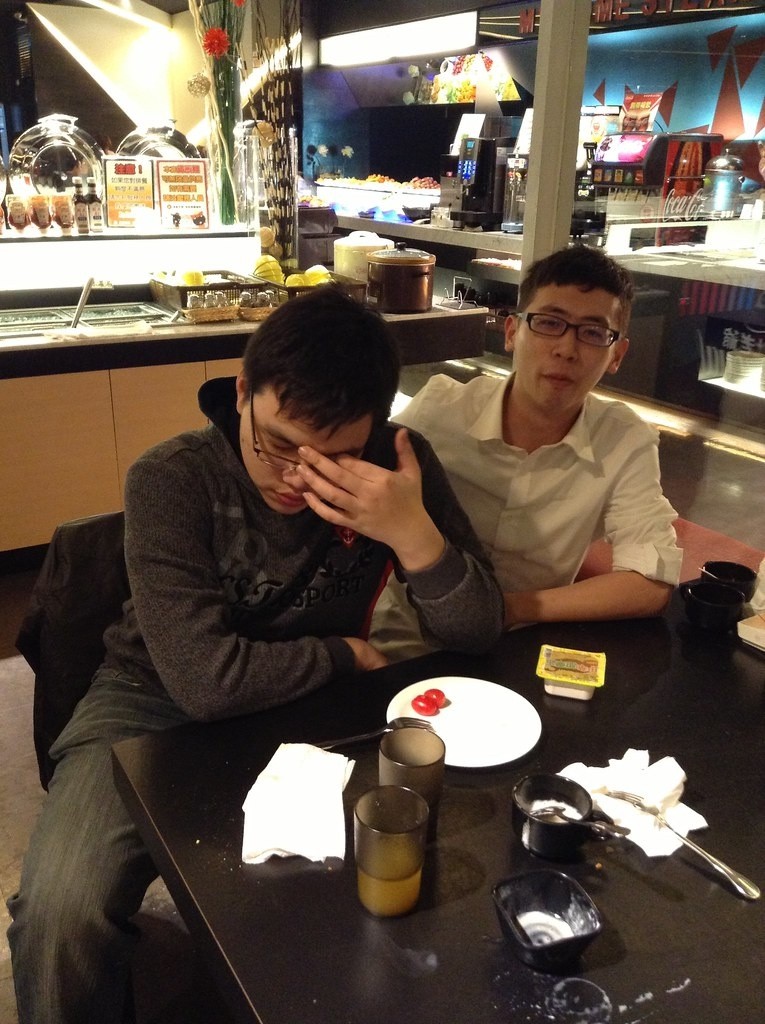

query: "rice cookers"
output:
334 230 394 283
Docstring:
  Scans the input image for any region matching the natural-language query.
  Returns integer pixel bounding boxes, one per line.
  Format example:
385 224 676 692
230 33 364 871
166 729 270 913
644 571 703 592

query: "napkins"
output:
554 749 710 857
242 743 356 864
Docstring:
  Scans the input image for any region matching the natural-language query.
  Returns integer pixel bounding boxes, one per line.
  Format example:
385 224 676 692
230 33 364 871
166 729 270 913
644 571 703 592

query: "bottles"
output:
215 292 223 307
266 290 274 306
241 292 253 307
255 292 268 307
187 295 200 308
204 294 214 307
84 177 105 233
71 176 90 233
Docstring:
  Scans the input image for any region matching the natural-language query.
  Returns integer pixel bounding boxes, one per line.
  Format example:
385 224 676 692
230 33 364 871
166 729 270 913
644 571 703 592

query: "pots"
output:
366 242 437 313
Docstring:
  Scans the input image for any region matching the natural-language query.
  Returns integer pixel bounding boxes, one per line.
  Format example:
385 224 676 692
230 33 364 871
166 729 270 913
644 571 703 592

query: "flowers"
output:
402 65 420 105
186 0 248 122
307 145 353 166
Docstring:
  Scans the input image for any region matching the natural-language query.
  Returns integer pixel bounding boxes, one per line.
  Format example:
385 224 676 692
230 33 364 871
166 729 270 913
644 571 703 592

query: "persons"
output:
7 282 506 1024
369 242 683 663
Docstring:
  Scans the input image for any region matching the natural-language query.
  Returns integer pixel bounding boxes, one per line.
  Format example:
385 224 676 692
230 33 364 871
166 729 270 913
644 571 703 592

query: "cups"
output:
512 772 618 861
353 784 429 920
378 729 446 845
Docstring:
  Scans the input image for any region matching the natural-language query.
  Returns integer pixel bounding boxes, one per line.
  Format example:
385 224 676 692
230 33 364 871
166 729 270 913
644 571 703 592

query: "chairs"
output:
12 512 127 795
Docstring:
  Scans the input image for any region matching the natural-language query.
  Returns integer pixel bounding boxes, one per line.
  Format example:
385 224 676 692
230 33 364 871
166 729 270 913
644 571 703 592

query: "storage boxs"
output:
149 270 267 311
249 270 367 308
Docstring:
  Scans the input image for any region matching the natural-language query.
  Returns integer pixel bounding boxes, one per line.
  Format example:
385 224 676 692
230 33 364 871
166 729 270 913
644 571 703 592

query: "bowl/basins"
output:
702 560 759 603
491 868 605 974
255 254 333 286
402 206 431 221
679 581 746 633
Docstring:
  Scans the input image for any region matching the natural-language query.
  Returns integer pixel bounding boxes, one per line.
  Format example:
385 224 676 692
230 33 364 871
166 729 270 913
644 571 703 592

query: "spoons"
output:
530 806 631 840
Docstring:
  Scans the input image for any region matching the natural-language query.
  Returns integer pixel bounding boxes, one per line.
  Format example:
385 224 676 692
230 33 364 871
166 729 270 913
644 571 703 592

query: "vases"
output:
245 135 298 272
312 166 344 180
198 55 242 227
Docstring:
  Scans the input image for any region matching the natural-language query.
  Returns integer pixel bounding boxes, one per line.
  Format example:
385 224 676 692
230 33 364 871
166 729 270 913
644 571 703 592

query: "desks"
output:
110 576 765 1024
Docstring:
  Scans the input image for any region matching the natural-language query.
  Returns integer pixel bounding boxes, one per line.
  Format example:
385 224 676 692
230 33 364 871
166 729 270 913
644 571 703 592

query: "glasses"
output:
248 387 302 471
515 312 620 348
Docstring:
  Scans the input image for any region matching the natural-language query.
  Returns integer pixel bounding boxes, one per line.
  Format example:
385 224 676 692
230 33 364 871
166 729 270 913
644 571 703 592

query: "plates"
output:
723 350 765 393
386 675 542 769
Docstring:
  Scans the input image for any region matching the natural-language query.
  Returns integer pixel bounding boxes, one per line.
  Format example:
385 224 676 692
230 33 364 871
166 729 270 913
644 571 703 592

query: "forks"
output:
312 716 435 754
608 791 760 901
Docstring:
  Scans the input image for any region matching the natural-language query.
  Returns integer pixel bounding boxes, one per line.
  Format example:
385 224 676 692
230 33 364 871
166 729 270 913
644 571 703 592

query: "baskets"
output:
242 306 277 322
181 305 239 323
